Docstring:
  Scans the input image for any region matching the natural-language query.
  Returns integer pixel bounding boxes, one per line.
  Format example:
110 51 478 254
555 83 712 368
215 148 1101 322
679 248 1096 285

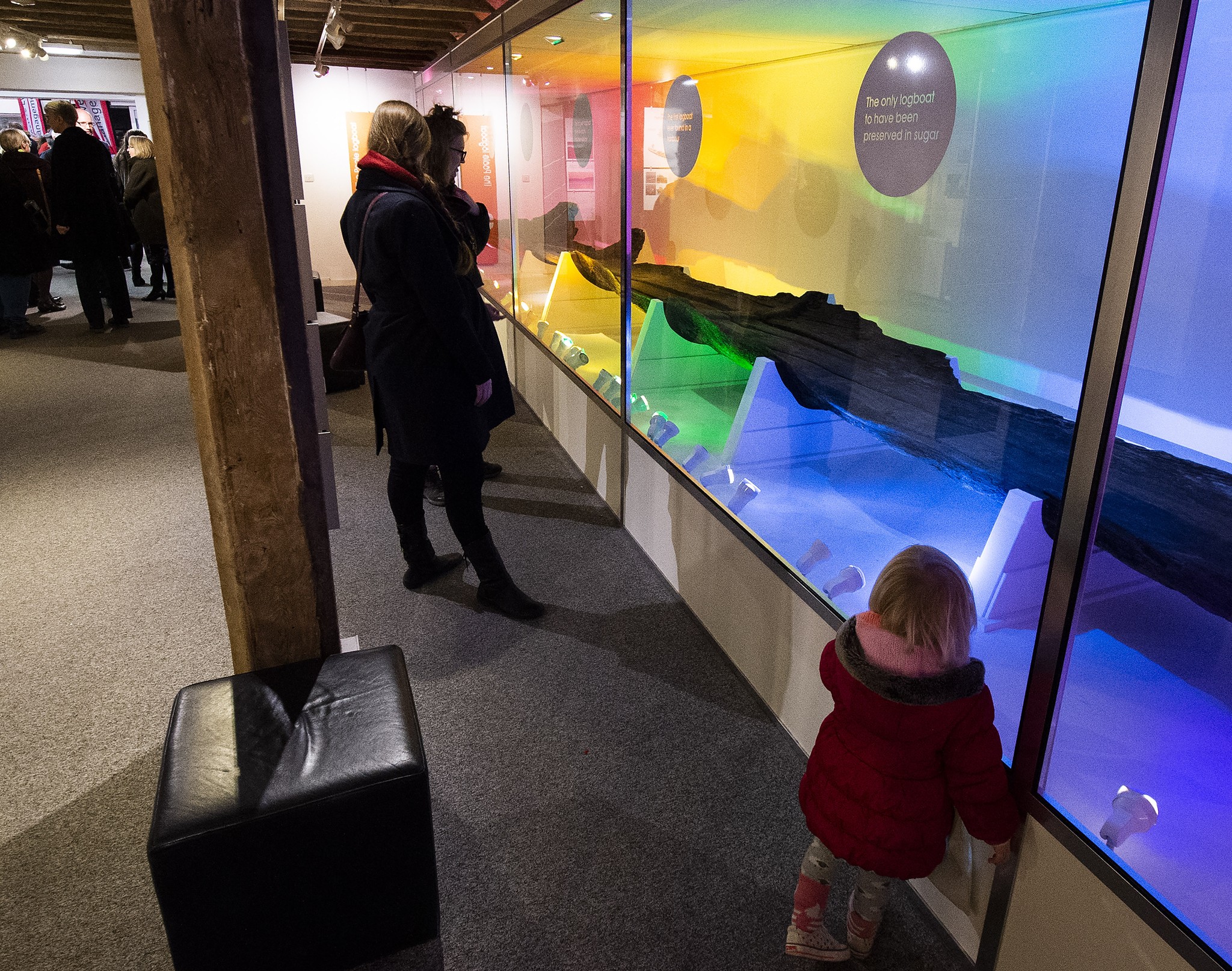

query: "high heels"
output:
141 286 166 301
166 286 175 298
38 295 66 313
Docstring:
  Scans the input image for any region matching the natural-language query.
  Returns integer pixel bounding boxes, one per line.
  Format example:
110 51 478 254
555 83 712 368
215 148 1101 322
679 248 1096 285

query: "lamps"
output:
1100 784 1159 852
648 415 666 440
0 22 83 60
728 477 761 515
593 369 621 411
549 330 588 369
699 464 734 487
797 540 834 575
499 292 538 326
479 267 499 292
631 395 650 415
683 445 709 473
537 321 549 339
654 420 680 446
313 0 355 77
822 565 866 601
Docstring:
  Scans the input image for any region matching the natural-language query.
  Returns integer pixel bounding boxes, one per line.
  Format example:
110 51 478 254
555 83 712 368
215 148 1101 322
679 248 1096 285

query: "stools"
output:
312 311 366 393
146 642 441 971
312 270 324 313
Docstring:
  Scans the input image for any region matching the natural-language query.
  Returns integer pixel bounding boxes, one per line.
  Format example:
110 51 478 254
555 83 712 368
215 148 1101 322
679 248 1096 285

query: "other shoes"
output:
10 321 44 340
60 263 75 270
108 317 130 326
402 552 464 590
476 583 546 619
89 324 104 333
53 296 61 302
131 275 146 287
120 257 132 271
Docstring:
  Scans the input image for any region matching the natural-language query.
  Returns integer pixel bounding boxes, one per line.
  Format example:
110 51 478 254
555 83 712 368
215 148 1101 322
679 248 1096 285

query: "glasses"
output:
449 147 467 162
128 145 133 149
76 121 96 128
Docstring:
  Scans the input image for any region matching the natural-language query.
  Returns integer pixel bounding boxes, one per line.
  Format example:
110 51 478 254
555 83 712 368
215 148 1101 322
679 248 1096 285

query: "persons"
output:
419 104 507 505
339 99 545 621
784 542 1021 963
0 99 176 340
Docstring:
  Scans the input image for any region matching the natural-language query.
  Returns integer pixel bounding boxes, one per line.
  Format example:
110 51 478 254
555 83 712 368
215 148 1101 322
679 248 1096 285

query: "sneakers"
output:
421 470 446 505
785 925 850 961
847 911 874 960
482 460 501 478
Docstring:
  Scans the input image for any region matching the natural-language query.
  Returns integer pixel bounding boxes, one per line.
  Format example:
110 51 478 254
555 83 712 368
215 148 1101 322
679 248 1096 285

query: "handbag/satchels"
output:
329 311 371 371
